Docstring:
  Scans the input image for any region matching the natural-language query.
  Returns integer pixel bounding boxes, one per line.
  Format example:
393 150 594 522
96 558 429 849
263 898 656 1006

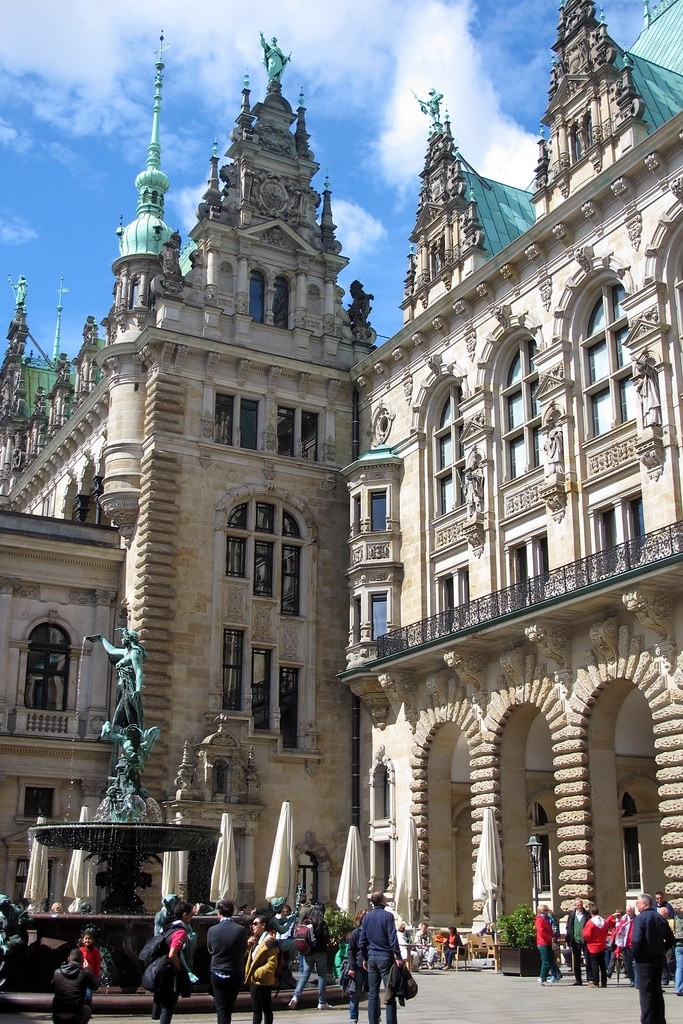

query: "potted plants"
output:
492 902 542 977
320 906 358 973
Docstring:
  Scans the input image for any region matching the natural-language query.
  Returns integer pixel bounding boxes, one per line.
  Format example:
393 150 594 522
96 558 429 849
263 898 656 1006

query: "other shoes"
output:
350 1017 381 1024
606 972 611 978
538 976 557 986
624 975 639 989
411 964 454 972
572 979 599 988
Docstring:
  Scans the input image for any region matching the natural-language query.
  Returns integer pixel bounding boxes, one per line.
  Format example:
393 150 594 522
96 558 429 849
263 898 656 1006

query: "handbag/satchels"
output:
552 938 559 950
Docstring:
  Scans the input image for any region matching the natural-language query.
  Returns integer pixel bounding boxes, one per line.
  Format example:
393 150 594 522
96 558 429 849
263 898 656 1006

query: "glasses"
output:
656 897 663 900
251 922 263 926
190 912 193 916
282 910 289 914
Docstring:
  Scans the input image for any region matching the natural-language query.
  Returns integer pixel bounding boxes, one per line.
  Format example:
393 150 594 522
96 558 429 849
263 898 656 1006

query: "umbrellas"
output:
472 808 503 944
64 804 92 899
23 815 48 904
335 824 368 921
209 813 238 916
264 801 299 914
162 822 178 898
395 819 423 929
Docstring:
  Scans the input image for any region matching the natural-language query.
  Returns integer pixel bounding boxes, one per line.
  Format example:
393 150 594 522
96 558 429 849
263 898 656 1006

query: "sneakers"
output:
288 994 333 1009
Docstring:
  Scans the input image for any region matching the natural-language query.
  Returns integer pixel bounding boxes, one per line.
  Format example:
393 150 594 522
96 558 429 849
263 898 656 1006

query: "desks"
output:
399 943 423 972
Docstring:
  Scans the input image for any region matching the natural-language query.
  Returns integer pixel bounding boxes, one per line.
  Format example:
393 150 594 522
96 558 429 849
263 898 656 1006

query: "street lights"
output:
525 836 543 914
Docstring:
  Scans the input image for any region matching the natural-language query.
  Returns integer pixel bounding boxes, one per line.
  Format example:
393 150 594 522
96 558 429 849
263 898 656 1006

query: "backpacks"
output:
138 924 187 968
293 918 323 956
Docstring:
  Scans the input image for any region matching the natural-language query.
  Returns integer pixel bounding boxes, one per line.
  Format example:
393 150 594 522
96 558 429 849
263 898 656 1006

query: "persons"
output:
534 891 683 996
271 896 298 987
11 275 28 313
50 930 101 1024
627 894 676 1024
151 891 418 1024
260 31 291 83
478 923 495 944
86 627 145 731
154 894 199 983
420 88 444 122
0 893 34 987
397 923 466 971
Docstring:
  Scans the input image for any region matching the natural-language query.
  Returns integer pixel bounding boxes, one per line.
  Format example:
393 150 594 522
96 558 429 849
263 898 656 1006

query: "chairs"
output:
411 928 505 972
